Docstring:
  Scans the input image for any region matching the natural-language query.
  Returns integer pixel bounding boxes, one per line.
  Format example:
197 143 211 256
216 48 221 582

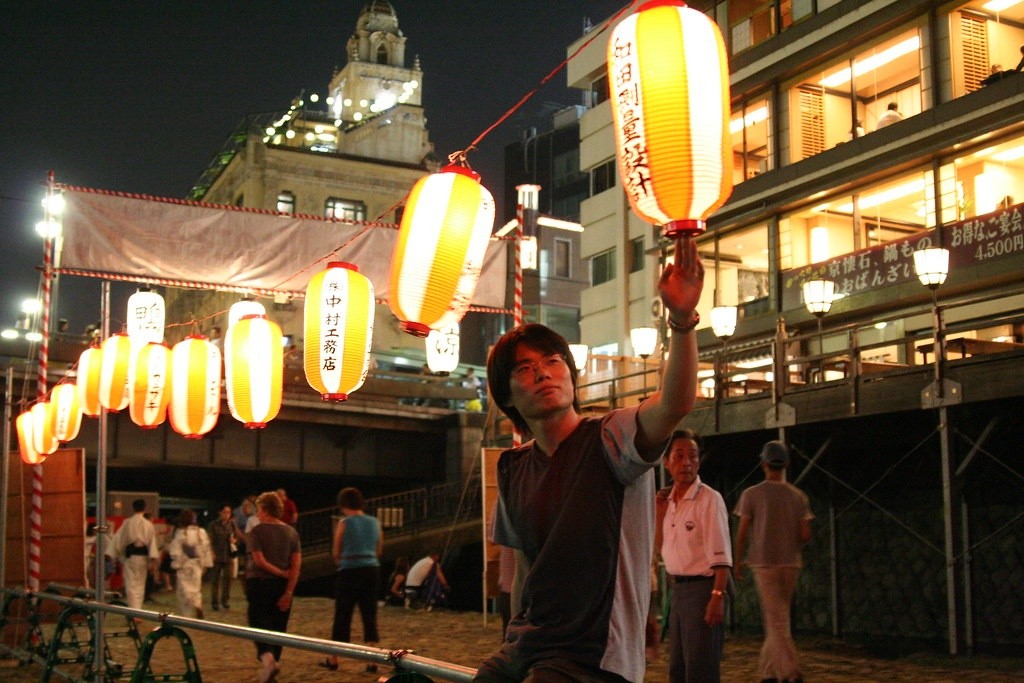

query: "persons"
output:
472 234 704 683
849 118 864 140
876 103 904 130
654 428 734 683
316 487 384 673
82 325 96 346
489 498 515 645
209 326 223 351
93 320 101 341
735 439 817 683
419 365 482 412
385 547 448 609
991 64 1003 73
55 319 68 341
83 490 298 623
245 491 302 683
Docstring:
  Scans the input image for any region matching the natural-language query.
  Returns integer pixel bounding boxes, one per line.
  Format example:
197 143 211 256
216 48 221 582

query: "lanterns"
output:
426 320 460 375
16 262 374 466
388 166 495 338
607 0 734 238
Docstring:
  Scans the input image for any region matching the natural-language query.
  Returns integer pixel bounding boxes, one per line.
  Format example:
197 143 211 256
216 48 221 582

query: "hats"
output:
760 441 788 466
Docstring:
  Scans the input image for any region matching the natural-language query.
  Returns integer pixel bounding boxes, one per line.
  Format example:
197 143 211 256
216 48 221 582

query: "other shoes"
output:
222 602 230 609
318 657 337 670
213 605 219 611
365 664 378 673
263 662 280 683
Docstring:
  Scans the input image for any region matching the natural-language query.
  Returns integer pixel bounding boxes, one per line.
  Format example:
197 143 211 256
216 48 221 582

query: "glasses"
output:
509 354 568 378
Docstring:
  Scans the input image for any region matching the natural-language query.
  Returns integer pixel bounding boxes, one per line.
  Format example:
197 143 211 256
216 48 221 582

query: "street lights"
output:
912 247 962 656
710 304 738 398
803 277 835 381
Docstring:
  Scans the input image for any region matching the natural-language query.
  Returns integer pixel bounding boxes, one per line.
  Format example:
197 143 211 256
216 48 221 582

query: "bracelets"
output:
711 589 727 598
285 591 293 594
668 310 700 333
